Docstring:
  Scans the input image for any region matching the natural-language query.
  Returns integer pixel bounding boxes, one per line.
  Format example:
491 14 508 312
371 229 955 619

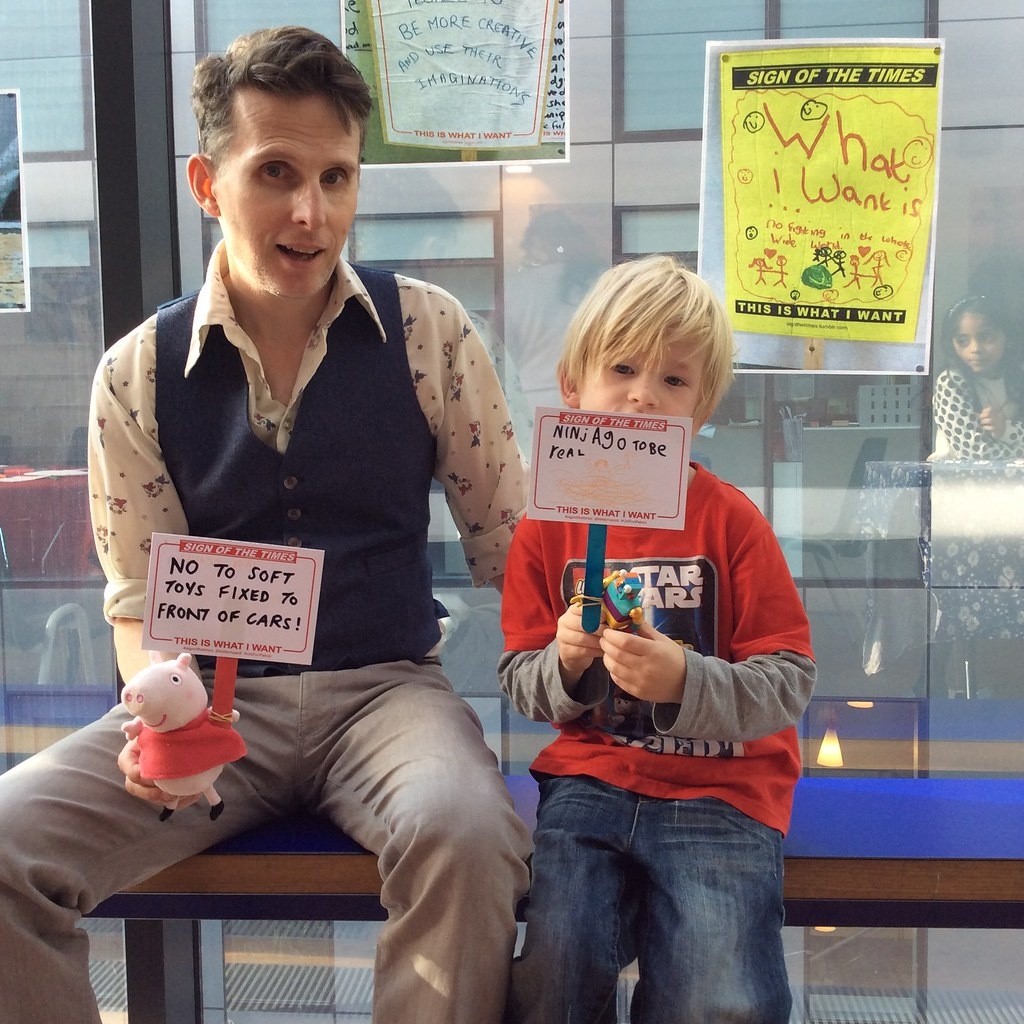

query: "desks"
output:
858 460 1024 697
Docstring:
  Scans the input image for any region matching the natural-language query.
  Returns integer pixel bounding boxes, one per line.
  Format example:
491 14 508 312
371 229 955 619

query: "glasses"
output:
955 330 997 347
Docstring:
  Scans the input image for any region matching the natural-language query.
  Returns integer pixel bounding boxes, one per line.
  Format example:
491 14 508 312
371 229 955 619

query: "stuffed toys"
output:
120 650 247 821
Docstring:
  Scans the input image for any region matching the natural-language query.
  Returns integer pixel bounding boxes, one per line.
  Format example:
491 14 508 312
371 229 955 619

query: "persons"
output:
0 25 530 1024
924 294 1024 464
496 255 817 1024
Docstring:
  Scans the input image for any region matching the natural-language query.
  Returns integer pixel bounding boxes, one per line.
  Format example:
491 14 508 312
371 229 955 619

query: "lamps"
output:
814 719 844 767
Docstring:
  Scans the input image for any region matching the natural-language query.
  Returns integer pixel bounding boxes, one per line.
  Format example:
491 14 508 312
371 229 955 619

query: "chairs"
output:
774 437 887 642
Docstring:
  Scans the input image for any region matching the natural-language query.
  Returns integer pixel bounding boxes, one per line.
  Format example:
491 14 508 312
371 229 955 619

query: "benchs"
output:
73 775 1024 1024
